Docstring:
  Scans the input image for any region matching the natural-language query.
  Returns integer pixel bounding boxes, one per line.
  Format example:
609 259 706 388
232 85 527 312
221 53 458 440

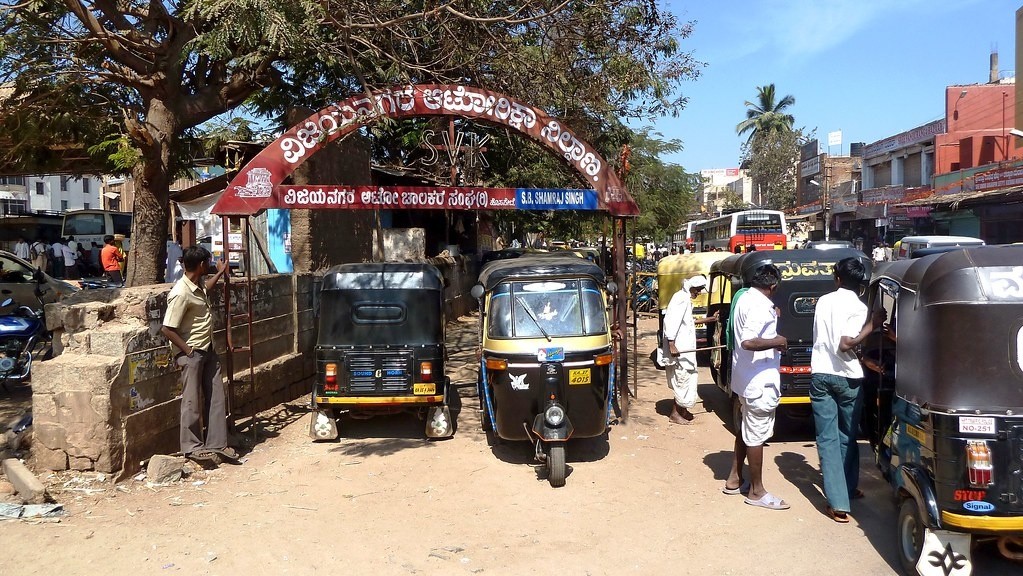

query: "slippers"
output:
723 479 750 494
824 505 849 521
209 447 239 459
744 492 789 509
849 489 863 497
186 448 214 460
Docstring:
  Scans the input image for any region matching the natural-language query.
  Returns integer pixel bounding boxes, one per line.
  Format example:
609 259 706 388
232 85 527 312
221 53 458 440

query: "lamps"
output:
956 91 968 103
904 148 908 159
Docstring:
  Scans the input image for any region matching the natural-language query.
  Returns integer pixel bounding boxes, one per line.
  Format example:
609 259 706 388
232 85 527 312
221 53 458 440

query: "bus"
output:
673 219 708 248
693 209 788 254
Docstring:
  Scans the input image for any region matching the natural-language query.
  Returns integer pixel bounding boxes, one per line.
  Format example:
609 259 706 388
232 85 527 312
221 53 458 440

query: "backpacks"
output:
30 243 41 260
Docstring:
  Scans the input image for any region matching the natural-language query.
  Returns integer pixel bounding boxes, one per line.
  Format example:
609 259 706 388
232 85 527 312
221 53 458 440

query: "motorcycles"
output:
78 274 125 291
705 247 872 437
861 241 1023 576
310 261 461 442
471 256 623 488
657 251 737 366
0 265 75 396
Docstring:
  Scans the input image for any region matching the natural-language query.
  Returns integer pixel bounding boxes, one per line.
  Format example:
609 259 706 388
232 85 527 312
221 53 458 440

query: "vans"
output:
61 209 133 254
896 236 985 260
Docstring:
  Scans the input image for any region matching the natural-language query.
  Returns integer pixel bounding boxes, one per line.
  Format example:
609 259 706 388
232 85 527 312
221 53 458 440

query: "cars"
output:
166 232 174 254
0 250 83 317
804 241 855 251
212 229 241 270
196 234 212 253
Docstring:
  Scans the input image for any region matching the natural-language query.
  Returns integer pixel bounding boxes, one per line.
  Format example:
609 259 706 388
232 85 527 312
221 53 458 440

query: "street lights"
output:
810 180 830 241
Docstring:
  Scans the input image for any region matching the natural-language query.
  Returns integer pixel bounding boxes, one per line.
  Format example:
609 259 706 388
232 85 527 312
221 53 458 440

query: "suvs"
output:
0 209 68 253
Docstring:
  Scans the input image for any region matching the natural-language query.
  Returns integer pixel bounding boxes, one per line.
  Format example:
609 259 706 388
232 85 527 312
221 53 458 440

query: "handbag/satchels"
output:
657 347 677 366
49 248 54 256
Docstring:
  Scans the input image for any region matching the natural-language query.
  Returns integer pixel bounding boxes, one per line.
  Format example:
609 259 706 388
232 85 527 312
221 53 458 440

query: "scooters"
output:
549 234 657 314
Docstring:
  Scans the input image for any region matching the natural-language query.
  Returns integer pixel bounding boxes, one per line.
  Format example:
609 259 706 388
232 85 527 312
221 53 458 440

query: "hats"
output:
689 276 707 288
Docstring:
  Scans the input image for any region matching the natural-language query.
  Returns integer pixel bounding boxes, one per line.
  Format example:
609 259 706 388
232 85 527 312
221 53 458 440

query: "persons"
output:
646 240 762 260
809 258 887 522
15 233 127 286
663 274 720 425
882 323 896 341
722 264 791 509
162 247 240 460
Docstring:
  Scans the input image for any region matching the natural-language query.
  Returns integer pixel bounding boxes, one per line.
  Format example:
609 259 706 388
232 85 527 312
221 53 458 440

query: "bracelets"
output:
186 350 192 356
